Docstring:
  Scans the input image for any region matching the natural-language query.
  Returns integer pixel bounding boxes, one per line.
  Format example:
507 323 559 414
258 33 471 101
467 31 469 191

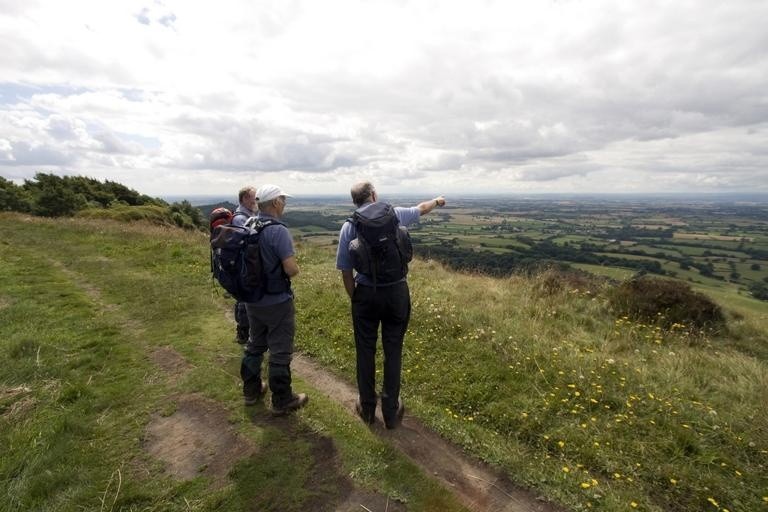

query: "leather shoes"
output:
356 394 375 423
272 392 308 417
245 382 268 406
386 394 403 429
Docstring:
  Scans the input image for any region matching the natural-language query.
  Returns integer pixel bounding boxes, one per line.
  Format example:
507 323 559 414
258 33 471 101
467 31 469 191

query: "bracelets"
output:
434 198 439 206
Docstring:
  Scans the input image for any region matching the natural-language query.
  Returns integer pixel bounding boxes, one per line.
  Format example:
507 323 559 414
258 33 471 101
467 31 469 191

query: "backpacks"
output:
347 200 413 283
211 219 293 303
209 207 250 231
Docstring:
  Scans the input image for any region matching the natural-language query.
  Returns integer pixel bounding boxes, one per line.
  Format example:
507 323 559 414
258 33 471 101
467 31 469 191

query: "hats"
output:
253 183 293 203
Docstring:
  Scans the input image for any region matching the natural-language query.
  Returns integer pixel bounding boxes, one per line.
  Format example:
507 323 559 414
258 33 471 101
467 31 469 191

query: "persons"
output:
336 179 447 431
232 185 260 347
238 184 308 417
